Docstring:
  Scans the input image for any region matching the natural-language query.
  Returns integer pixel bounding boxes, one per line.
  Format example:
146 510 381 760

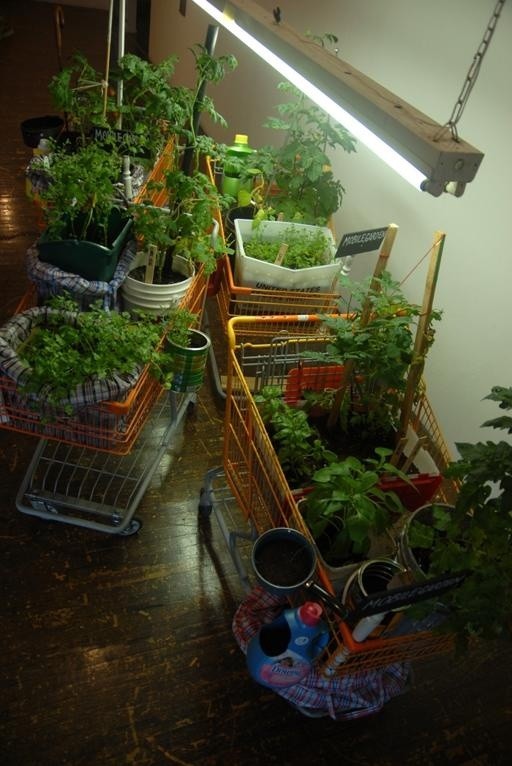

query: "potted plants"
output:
252 272 512 641
4 4 341 442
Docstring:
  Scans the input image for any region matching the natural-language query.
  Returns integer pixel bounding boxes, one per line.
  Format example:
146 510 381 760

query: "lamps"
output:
191 0 511 198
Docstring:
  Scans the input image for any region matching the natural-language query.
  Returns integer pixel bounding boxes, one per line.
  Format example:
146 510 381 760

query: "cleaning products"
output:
244 602 329 688
220 135 257 197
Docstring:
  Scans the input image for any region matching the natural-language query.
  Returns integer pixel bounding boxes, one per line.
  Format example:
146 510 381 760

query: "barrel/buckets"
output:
399 503 472 583
119 251 196 321
342 557 408 637
